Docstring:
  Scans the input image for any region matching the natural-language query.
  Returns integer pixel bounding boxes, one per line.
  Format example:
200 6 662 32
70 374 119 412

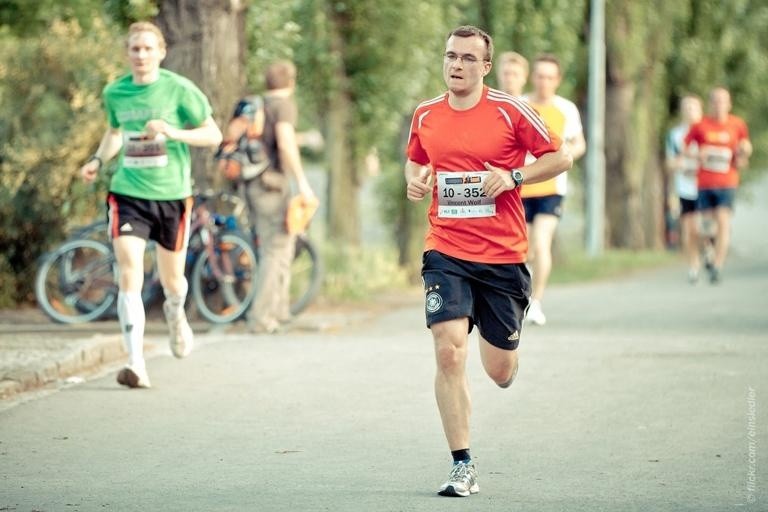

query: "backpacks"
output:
214 92 282 184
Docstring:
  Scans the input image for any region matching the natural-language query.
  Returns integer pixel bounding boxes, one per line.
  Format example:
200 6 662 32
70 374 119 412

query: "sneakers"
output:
496 348 519 389
269 315 321 335
436 456 480 498
687 244 722 285
526 302 546 326
163 298 195 359
117 360 152 389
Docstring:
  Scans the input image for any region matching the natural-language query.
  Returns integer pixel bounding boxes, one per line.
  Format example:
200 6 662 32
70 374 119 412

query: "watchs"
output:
511 168 524 188
87 155 103 169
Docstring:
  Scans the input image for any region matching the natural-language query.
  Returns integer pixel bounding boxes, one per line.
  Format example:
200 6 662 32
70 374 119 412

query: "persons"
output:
681 86 752 286
405 26 574 496
245 58 314 334
495 51 529 101
81 21 224 389
519 55 586 325
665 92 716 285
